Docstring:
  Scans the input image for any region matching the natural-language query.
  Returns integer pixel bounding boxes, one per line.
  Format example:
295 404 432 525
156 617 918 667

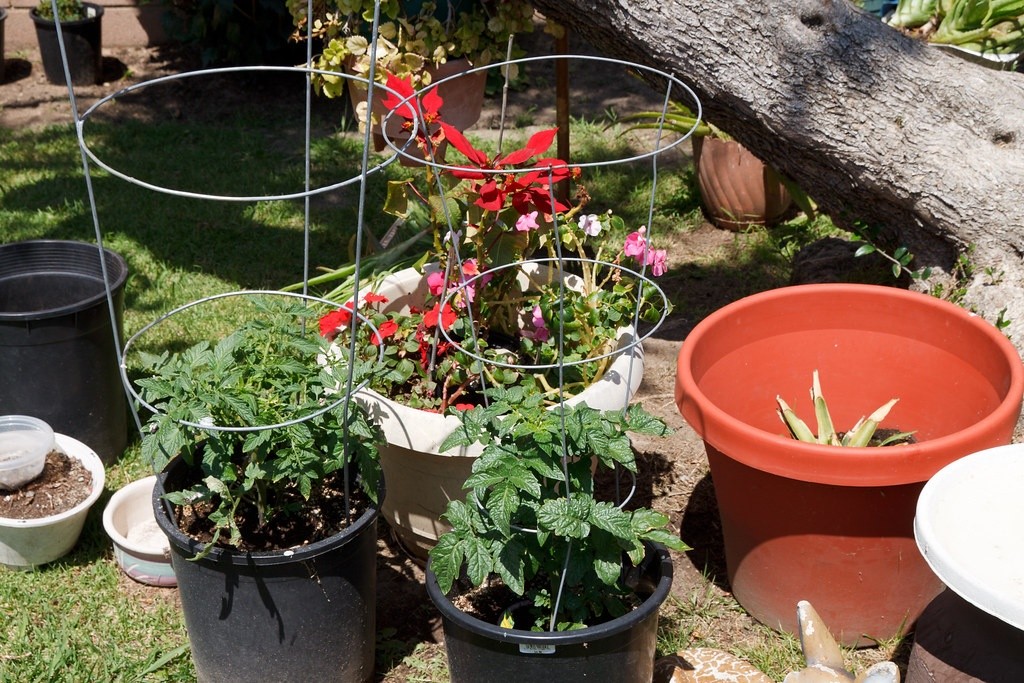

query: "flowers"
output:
318 72 673 416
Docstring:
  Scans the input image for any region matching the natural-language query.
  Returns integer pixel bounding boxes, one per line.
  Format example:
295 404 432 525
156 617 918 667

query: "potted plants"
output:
284 0 566 168
601 100 816 232
138 0 241 120
131 294 389 682
28 1 104 85
674 283 1024 650
425 321 692 683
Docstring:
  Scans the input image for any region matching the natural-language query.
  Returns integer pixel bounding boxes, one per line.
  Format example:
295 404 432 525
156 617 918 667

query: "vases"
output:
101 475 179 589
0 240 130 465
317 266 644 559
0 430 106 572
